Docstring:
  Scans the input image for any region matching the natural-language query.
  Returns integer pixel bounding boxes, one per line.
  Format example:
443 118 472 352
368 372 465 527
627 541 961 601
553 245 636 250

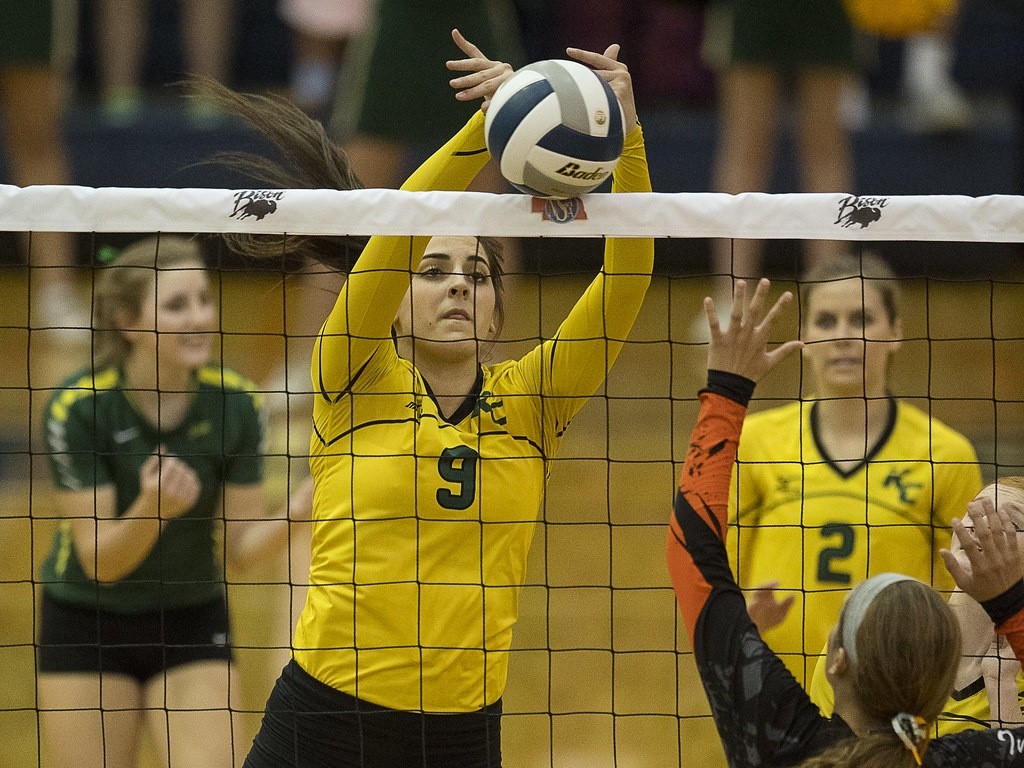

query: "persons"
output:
663 249 1023 768
35 232 309 768
347 0 528 283
236 28 657 768
683 1 972 351
94 0 230 123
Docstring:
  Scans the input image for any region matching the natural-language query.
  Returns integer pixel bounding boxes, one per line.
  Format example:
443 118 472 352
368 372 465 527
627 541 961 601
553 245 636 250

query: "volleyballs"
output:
484 59 626 200
